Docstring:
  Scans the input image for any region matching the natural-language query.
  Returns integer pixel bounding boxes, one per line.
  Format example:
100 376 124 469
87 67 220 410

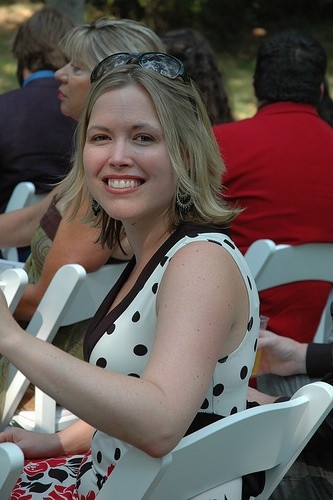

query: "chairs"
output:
2 182 46 261
93 378 333 500
4 261 131 434
243 238 333 344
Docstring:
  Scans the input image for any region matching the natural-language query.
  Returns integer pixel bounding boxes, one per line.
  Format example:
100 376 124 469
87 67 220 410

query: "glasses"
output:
89 53 198 121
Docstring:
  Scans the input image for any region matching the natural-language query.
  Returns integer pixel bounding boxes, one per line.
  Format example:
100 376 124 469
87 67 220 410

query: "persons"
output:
0 52 259 500
211 29 333 344
0 19 167 323
247 329 333 472
0 8 77 262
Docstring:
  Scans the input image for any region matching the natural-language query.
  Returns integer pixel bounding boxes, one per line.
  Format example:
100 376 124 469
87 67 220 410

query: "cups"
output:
250 316 269 378
